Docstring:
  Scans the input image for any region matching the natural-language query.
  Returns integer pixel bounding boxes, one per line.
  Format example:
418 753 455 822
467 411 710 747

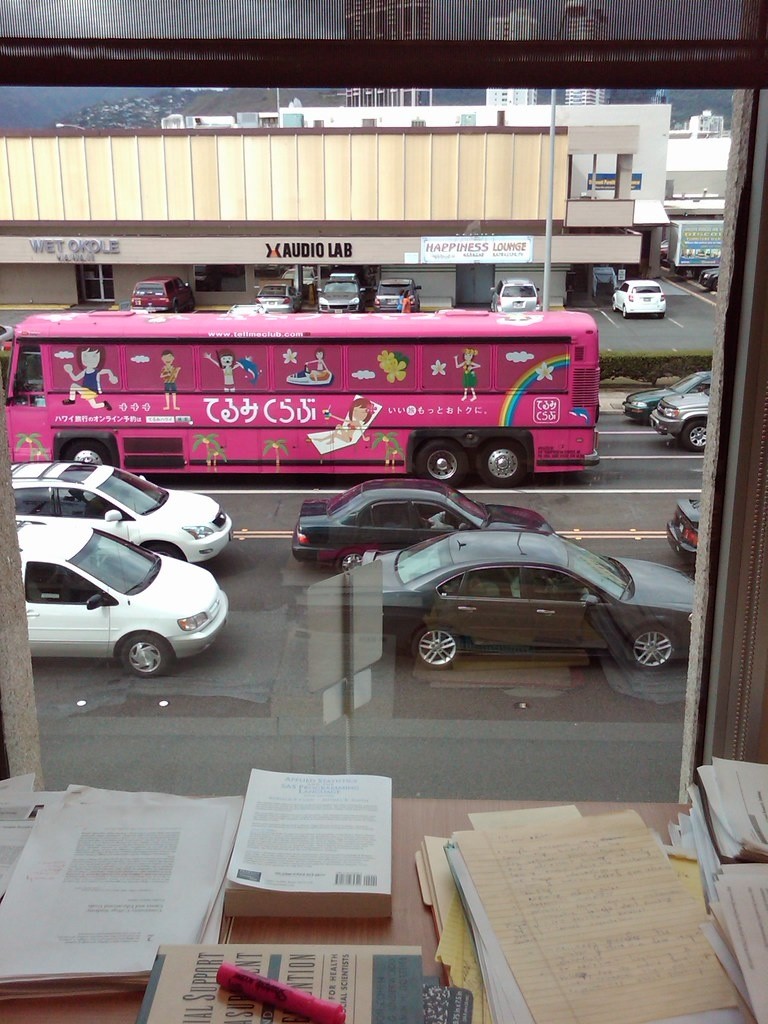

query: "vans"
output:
129 275 195 315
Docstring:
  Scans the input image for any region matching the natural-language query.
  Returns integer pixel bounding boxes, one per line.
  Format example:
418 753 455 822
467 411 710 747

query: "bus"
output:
4 306 605 491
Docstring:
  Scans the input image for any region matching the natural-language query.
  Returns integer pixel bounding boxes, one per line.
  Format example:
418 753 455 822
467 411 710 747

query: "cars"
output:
356 528 697 671
0 323 14 342
612 279 667 319
194 263 367 314
489 277 542 312
660 240 671 260
13 520 230 679
621 371 714 420
666 498 700 566
291 478 556 577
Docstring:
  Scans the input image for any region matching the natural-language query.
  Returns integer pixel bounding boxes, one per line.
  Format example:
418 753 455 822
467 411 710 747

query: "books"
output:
224 769 392 918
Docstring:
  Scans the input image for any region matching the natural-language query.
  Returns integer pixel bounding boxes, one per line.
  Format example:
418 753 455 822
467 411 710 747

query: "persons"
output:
254 298 264 309
397 288 411 313
420 507 454 531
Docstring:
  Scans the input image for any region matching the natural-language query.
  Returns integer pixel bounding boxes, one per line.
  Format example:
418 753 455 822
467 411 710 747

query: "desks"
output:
0 797 692 1024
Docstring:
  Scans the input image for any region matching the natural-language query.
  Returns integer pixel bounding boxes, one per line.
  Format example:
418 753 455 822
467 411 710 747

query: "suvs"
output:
648 386 711 452
699 268 720 291
372 278 423 315
7 460 233 563
